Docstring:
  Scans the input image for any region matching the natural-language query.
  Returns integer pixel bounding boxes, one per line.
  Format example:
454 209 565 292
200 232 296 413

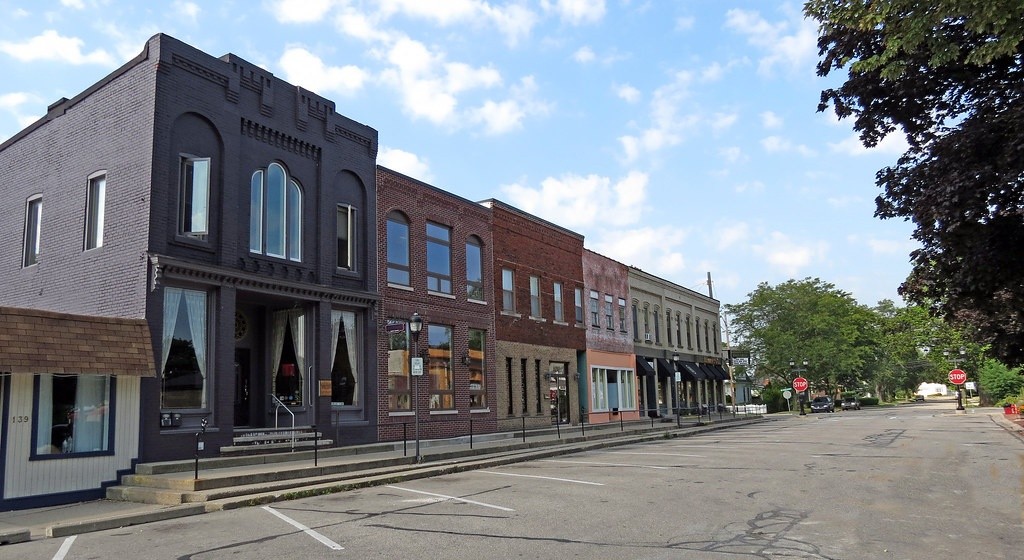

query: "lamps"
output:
545 372 550 380
462 352 470 367
574 372 580 381
421 348 430 364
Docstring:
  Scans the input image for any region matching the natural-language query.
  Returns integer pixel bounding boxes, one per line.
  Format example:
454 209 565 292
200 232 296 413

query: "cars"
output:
810 394 834 412
840 396 861 412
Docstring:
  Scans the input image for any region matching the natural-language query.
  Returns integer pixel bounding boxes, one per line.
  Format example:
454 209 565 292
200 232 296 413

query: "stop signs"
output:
791 376 808 391
949 368 968 385
